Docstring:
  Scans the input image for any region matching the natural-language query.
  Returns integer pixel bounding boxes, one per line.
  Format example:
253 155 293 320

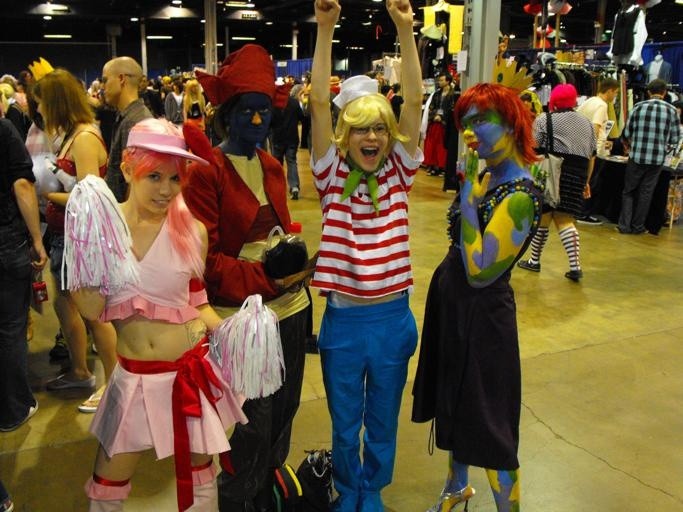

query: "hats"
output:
550 84 577 113
127 131 208 166
195 43 292 108
332 74 377 109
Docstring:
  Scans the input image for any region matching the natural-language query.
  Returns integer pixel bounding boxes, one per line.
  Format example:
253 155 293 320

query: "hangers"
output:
555 62 628 77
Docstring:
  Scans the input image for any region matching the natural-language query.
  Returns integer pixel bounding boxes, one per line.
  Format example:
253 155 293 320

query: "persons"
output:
1 0 682 280
0 1 423 511
415 83 559 511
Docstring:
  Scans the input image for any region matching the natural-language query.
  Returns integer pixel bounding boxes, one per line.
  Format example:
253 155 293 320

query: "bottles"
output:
280 221 308 293
43 157 77 192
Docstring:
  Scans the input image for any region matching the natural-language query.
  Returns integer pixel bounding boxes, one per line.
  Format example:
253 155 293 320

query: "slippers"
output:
78 394 101 413
46 376 95 389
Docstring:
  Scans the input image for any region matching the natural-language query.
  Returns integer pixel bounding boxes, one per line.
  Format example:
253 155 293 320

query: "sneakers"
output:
575 215 602 225
564 271 582 278
516 259 539 272
0 400 38 432
289 188 299 200
48 337 68 356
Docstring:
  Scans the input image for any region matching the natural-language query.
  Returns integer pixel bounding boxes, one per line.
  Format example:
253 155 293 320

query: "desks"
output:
589 154 683 233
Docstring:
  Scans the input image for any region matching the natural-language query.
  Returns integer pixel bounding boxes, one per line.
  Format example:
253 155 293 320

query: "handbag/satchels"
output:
527 154 563 209
296 449 332 508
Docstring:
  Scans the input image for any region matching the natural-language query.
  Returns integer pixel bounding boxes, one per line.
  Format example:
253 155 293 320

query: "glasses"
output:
352 123 386 136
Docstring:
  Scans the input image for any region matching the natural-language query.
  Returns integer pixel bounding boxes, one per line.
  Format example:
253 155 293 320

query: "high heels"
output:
427 485 475 512
